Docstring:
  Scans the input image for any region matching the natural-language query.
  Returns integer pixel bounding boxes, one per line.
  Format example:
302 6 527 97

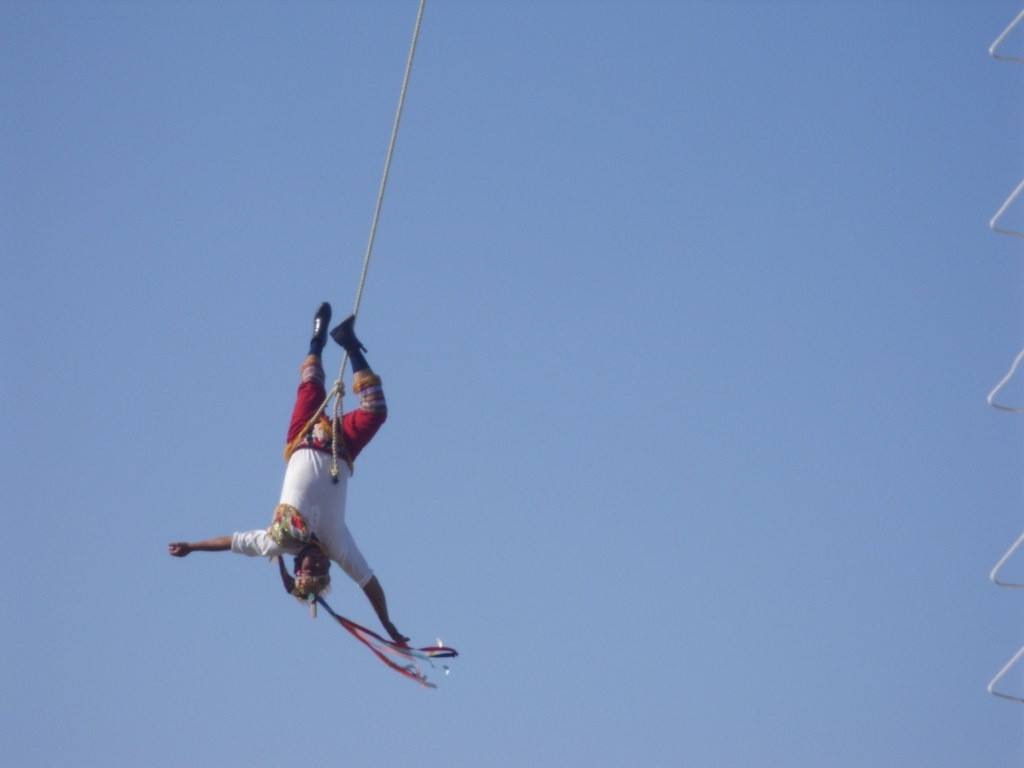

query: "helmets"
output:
295 575 332 599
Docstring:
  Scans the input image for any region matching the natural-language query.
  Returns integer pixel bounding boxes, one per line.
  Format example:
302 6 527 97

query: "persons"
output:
164 299 413 656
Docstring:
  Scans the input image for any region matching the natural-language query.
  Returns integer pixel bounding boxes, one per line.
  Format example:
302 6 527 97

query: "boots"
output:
329 314 362 352
310 303 332 349
